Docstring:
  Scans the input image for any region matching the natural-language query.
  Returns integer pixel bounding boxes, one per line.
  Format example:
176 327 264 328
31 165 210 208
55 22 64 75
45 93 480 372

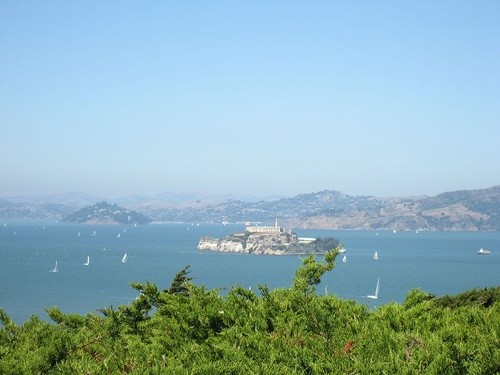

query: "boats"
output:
476 247 491 255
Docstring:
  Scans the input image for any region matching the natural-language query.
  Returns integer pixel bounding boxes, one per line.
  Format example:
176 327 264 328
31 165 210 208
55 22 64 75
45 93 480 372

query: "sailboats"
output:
373 252 379 259
46 222 140 274
343 256 348 263
367 277 381 300
